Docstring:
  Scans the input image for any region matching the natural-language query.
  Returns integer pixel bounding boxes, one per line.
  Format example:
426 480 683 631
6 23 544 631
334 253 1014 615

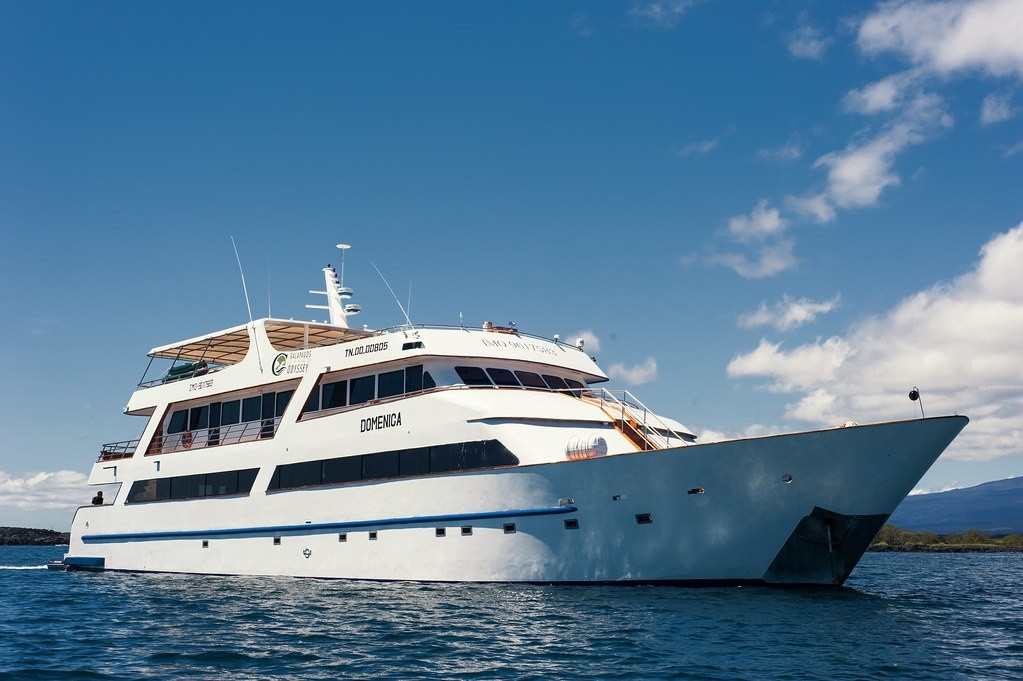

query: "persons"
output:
92 491 103 506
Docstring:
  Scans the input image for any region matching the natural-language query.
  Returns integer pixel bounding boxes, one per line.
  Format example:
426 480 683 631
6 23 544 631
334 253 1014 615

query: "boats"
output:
64 235 971 587
47 561 65 571
